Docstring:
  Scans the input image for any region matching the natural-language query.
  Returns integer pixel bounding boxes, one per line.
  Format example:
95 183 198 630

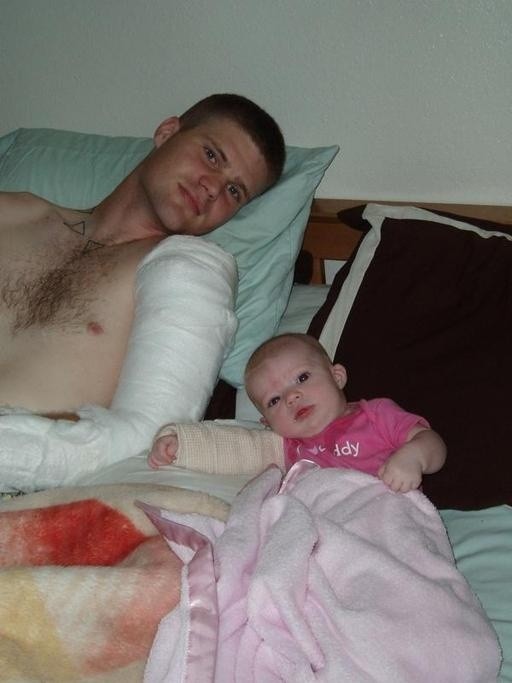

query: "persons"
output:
1 93 286 495
147 332 450 493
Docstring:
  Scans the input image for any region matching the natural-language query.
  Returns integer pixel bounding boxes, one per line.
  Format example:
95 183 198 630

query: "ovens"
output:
0 196 512 683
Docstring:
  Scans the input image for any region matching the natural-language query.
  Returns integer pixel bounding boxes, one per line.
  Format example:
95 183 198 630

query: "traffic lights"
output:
306 202 512 512
0 128 340 391
